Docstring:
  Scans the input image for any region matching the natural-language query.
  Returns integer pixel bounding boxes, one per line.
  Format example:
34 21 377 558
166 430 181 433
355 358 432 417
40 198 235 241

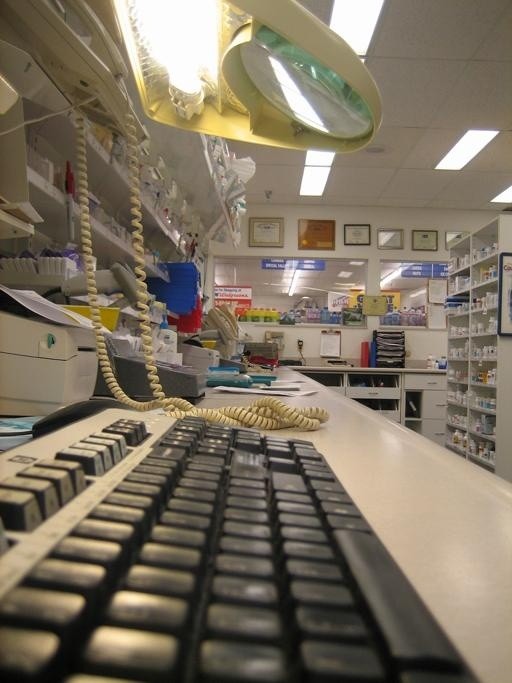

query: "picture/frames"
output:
446 231 461 243
412 229 438 251
249 217 284 247
298 218 335 250
497 250 512 338
344 224 370 245
376 228 403 250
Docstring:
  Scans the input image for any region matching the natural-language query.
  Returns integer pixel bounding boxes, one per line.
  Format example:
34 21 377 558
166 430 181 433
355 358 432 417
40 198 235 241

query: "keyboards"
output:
1 404 474 677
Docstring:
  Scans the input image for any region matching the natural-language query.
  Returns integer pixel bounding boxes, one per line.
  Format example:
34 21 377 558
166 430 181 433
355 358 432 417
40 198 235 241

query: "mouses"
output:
33 399 136 436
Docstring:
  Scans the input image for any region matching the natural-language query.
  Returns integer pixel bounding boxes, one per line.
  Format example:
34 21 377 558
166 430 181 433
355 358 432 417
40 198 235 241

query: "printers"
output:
2 302 104 416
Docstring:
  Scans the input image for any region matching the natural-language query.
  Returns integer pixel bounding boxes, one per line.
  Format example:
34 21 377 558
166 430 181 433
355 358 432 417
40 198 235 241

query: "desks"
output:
191 367 512 683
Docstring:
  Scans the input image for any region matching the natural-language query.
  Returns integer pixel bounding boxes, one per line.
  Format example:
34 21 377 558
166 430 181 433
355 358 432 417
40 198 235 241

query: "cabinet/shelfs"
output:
0 0 238 332
290 366 447 443
445 215 512 484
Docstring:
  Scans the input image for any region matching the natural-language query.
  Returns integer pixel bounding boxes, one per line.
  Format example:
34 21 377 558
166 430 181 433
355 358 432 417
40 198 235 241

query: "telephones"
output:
2 0 151 149
208 304 268 373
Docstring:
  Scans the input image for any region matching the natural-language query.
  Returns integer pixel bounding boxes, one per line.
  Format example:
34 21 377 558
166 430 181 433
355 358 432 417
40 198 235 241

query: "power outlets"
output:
296 338 305 349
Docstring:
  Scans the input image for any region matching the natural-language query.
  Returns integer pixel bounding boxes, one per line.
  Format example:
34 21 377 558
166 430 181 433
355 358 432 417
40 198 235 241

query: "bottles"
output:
306 306 340 324
383 306 425 326
426 354 445 369
246 307 278 322
444 243 496 468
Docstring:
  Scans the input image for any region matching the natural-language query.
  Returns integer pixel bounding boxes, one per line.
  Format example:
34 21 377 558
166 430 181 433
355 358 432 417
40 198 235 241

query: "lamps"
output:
113 1 383 154
